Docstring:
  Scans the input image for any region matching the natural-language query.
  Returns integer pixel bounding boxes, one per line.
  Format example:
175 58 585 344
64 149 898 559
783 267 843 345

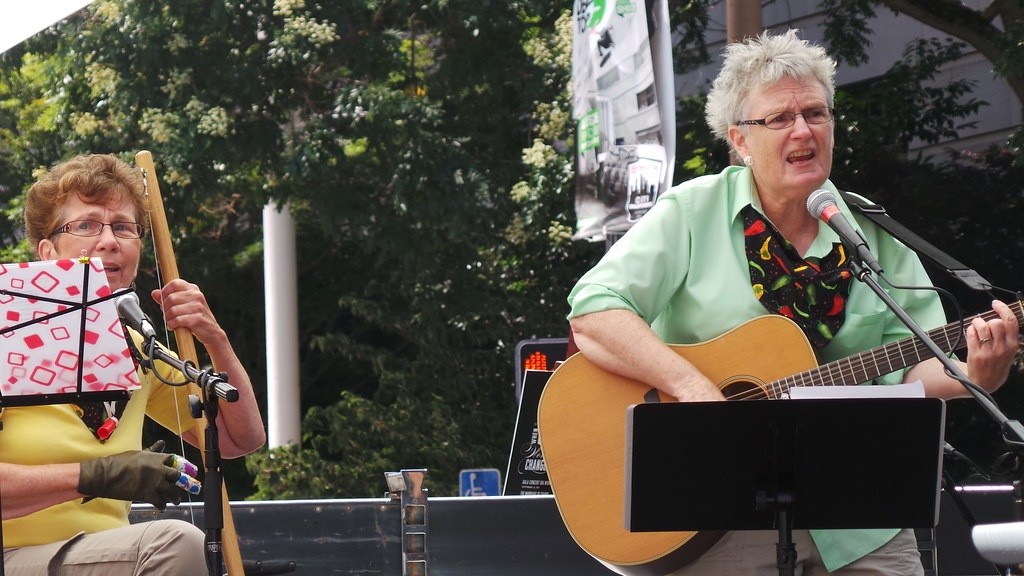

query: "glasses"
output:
48 220 145 239
732 107 833 130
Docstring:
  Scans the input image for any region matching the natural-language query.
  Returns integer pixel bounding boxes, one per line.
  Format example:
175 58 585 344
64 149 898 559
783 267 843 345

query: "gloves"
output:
77 440 202 510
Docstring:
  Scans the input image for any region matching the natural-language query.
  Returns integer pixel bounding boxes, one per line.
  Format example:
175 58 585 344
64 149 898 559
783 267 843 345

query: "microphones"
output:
113 287 157 340
807 188 885 277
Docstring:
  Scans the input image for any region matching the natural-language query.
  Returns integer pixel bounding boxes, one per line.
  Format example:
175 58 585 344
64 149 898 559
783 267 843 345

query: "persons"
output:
0 155 265 576
565 30 1019 576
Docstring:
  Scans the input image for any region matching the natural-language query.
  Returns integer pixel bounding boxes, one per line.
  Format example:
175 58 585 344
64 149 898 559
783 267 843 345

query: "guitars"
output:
535 298 1023 576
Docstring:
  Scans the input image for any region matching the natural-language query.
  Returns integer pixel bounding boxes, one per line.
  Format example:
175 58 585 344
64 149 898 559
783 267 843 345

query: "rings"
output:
980 337 991 343
